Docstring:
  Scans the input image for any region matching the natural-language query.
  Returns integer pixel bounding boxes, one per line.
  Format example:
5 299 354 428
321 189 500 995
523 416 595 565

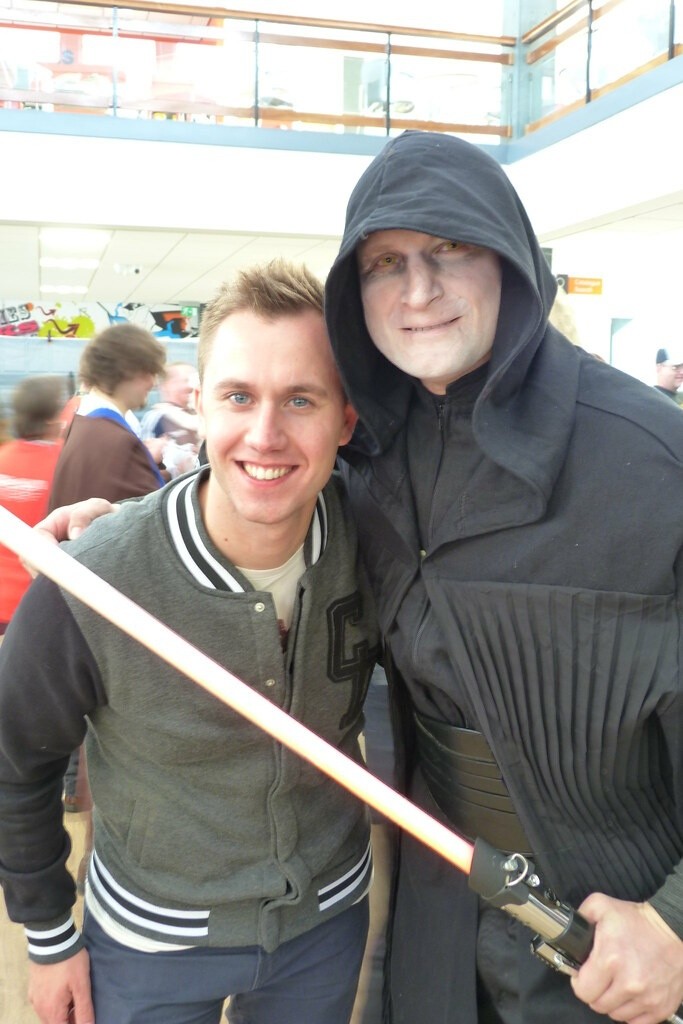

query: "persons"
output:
62 380 202 482
142 366 201 447
652 345 683 408
0 374 82 813
47 323 167 892
19 129 683 1024
0 259 386 1024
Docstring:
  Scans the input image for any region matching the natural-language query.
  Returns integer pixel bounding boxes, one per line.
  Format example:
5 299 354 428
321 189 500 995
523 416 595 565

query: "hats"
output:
656 349 683 366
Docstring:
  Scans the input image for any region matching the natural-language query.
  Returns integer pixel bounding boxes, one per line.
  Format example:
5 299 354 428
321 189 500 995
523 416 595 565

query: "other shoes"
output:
64 793 92 812
